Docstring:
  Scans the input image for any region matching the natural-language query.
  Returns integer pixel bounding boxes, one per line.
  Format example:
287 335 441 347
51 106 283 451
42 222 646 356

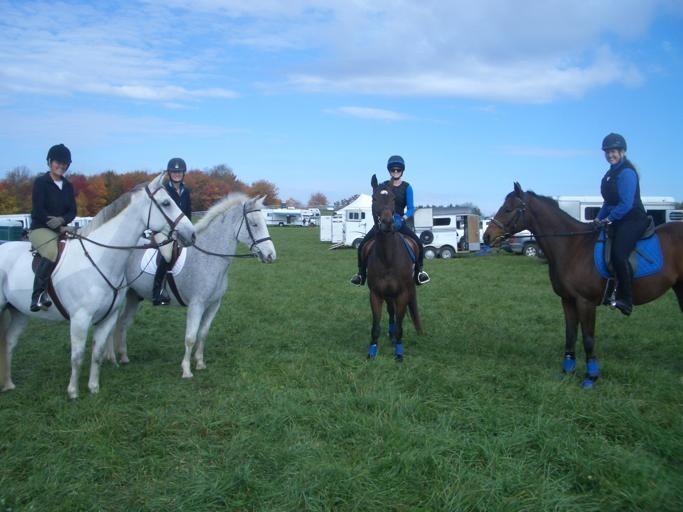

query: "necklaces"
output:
350 155 429 284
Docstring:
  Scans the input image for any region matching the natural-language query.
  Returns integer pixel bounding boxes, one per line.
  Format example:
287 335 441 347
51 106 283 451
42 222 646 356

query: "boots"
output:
30 256 54 311
350 247 365 285
415 245 428 285
608 260 634 316
152 264 171 305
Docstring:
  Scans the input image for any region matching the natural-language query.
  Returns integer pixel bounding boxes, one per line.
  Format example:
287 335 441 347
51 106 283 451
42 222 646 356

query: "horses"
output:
103 190 277 382
0 170 196 400
366 173 424 364
482 179 683 389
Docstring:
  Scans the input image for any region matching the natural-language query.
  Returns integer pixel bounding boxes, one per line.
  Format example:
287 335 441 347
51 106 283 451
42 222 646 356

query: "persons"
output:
27 145 76 311
593 133 649 317
150 157 190 306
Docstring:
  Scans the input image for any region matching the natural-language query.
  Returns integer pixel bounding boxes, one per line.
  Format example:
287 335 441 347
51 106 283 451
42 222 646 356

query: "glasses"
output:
390 167 401 172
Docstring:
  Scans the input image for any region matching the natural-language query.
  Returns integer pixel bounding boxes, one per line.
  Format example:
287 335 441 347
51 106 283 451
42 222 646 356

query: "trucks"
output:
0 213 91 245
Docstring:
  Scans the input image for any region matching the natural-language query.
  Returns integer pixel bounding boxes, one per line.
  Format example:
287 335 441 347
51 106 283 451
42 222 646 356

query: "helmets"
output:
168 157 185 172
602 132 626 151
46 145 72 162
387 155 404 168
